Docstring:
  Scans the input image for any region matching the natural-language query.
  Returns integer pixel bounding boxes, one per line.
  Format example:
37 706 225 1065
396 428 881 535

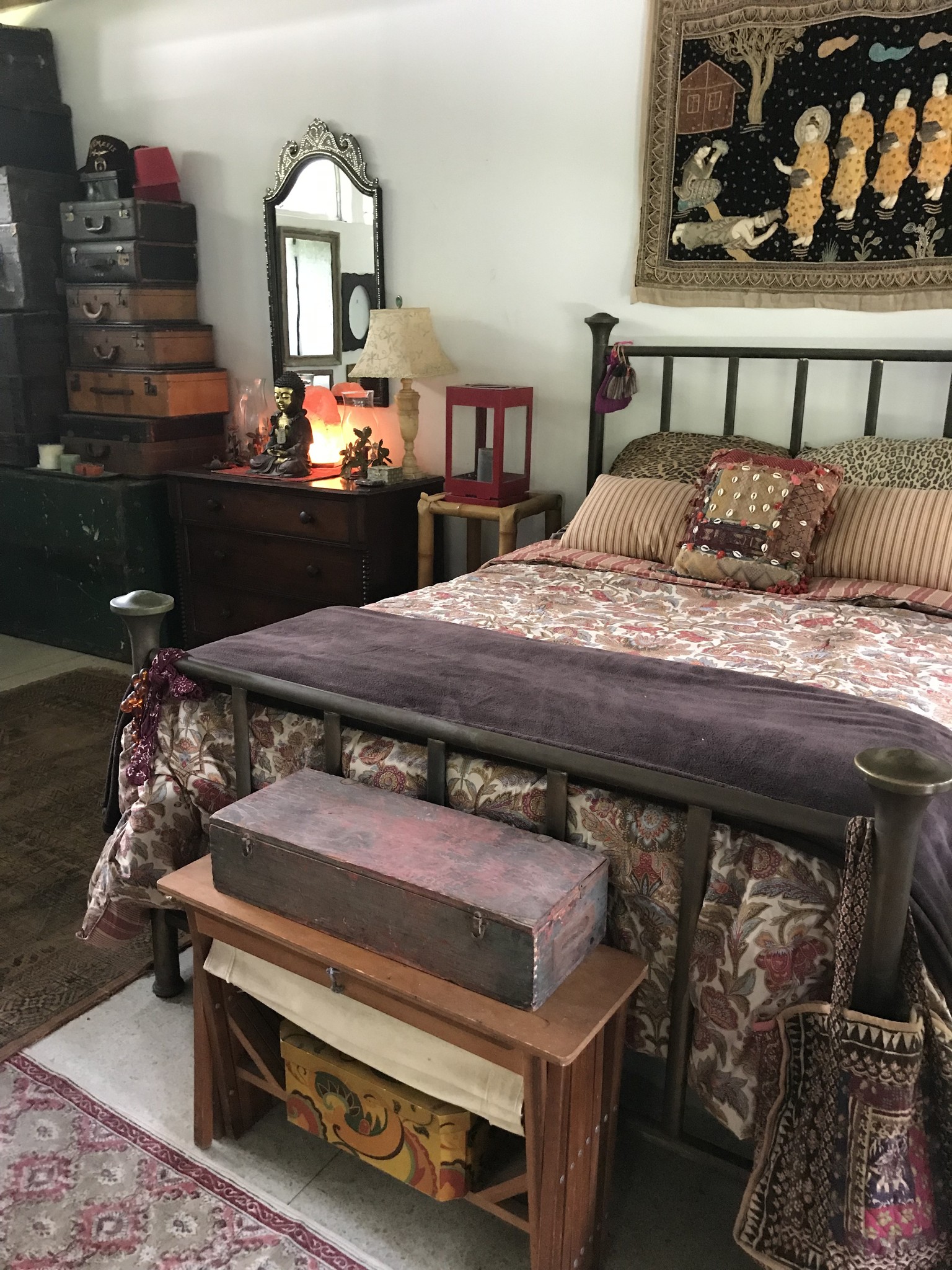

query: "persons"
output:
249 371 314 478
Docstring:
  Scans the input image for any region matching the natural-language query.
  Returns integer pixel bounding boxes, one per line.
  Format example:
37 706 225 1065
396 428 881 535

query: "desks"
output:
0 465 175 663
417 491 563 589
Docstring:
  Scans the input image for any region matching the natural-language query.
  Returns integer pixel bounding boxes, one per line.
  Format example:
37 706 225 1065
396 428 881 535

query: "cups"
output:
477 447 493 484
36 442 104 477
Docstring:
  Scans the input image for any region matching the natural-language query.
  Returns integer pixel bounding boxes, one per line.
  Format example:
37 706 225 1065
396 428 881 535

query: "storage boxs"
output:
80 170 133 201
208 767 608 1015
280 1019 499 1202
0 22 80 470
368 465 403 485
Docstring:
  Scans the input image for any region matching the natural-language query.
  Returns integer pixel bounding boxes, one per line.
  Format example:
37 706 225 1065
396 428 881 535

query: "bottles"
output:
234 377 270 461
340 389 380 473
296 372 314 388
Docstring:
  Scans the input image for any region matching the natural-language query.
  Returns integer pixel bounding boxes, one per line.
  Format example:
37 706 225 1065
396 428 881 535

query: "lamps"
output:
348 307 457 482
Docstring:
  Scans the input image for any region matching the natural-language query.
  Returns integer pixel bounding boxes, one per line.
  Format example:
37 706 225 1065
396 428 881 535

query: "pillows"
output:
556 472 952 593
794 435 952 490
609 431 793 486
670 448 845 595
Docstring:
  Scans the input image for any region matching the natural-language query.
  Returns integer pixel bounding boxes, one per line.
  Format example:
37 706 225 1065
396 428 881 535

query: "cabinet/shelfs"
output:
156 854 650 1270
165 460 444 650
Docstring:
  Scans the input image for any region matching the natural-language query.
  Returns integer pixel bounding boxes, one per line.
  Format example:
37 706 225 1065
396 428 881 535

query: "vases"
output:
233 377 269 462
339 390 382 481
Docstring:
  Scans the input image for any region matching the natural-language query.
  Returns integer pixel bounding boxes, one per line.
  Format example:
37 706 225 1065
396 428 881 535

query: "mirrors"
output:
261 114 390 408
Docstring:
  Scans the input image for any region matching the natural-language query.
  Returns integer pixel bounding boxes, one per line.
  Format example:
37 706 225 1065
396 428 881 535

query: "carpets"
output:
0 663 193 1059
0 1052 392 1270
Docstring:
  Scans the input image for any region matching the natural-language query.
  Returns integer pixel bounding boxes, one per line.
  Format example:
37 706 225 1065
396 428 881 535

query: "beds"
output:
81 310 952 1269
